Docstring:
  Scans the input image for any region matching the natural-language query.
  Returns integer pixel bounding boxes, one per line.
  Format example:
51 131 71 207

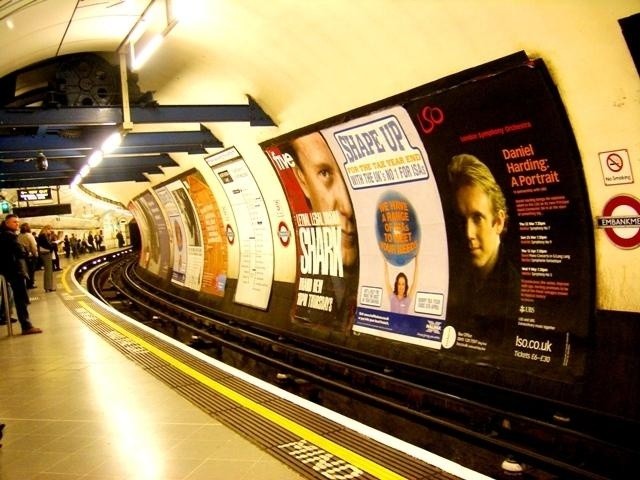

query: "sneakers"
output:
22 328 42 335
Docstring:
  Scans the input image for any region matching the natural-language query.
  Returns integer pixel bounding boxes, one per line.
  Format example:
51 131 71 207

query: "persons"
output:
276 129 360 333
442 151 522 346
378 248 422 317
1 212 127 337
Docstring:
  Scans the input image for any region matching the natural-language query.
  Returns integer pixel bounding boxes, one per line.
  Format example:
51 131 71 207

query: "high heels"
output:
46 289 56 292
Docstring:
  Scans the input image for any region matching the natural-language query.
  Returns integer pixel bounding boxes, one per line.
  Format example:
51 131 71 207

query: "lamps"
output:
67 123 136 220
31 150 49 172
124 1 178 76
